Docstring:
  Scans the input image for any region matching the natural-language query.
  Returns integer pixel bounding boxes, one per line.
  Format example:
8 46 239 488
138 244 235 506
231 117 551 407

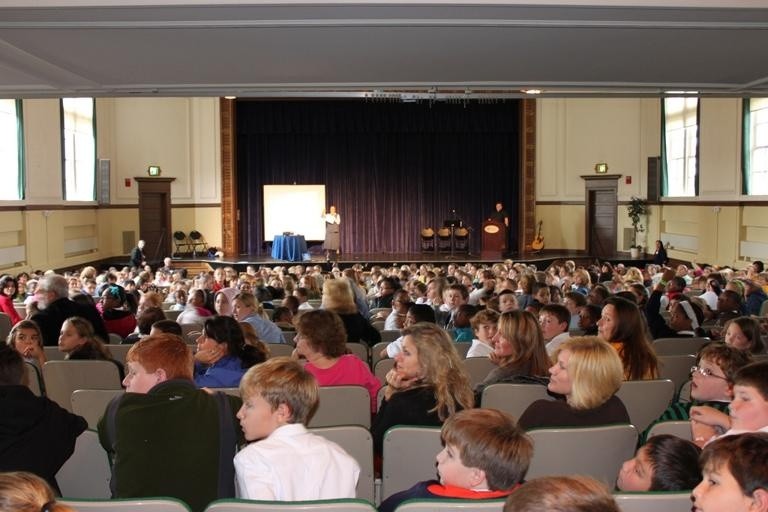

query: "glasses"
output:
690 366 733 381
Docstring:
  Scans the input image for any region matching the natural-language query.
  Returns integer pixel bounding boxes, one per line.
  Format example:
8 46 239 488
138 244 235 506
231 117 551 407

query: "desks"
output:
271 234 307 261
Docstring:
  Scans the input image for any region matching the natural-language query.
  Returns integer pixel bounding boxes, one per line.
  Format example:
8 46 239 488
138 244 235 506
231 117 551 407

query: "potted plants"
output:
626 194 652 257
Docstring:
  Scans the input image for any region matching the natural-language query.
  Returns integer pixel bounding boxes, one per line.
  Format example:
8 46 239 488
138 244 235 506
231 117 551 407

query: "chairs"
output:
188 231 210 257
435 224 454 258
451 223 474 257
419 226 436 256
172 230 201 258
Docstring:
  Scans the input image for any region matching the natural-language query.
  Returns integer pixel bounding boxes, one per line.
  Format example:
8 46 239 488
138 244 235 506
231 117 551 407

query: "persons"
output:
4 261 768 509
691 431 767 512
131 240 147 264
517 335 630 427
372 322 476 448
233 355 361 502
490 202 509 230
97 337 221 512
376 407 532 512
651 240 666 264
322 205 341 260
